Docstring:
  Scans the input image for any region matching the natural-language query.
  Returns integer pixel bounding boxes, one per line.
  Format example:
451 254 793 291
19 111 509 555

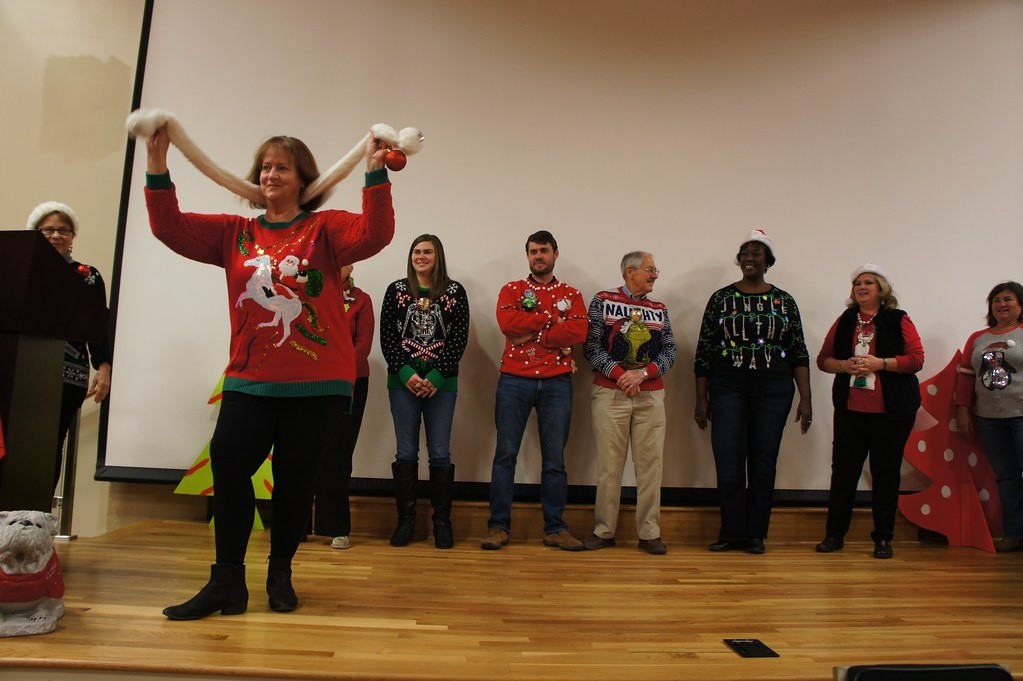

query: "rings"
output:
854 361 857 365
808 421 811 422
378 147 381 149
430 387 433 389
957 430 960 431
696 417 699 418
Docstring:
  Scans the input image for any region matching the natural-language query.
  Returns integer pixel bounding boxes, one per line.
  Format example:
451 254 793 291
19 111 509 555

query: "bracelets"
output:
883 358 887 369
641 368 648 380
840 360 842 372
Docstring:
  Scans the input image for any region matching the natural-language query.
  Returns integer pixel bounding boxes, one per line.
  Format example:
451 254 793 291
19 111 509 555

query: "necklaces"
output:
857 313 878 325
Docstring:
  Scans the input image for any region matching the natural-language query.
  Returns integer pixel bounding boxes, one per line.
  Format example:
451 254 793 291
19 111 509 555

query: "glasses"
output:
631 266 659 275
39 226 73 235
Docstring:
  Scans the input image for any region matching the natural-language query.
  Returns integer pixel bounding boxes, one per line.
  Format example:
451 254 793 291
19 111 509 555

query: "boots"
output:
267 558 297 612
429 463 456 548
390 461 418 547
162 563 249 619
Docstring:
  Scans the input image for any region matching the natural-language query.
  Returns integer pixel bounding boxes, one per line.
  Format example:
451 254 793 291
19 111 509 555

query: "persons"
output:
582 250 677 554
954 280 1023 553
816 265 925 559
380 233 470 550
694 226 812 554
144 125 396 620
299 267 375 549
28 201 115 491
479 231 589 552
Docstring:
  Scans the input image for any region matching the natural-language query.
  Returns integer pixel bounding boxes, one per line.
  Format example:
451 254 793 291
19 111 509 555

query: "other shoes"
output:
584 533 616 549
543 530 584 550
482 528 510 548
639 539 667 553
994 537 1023 552
331 536 351 549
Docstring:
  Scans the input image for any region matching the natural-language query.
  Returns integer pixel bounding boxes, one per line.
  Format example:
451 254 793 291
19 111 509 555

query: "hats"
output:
27 201 78 235
740 228 774 257
851 263 892 286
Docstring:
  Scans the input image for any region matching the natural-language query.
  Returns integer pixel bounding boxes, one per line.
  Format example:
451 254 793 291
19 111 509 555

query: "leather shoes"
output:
816 536 845 551
749 539 764 553
709 541 746 552
874 539 893 558
